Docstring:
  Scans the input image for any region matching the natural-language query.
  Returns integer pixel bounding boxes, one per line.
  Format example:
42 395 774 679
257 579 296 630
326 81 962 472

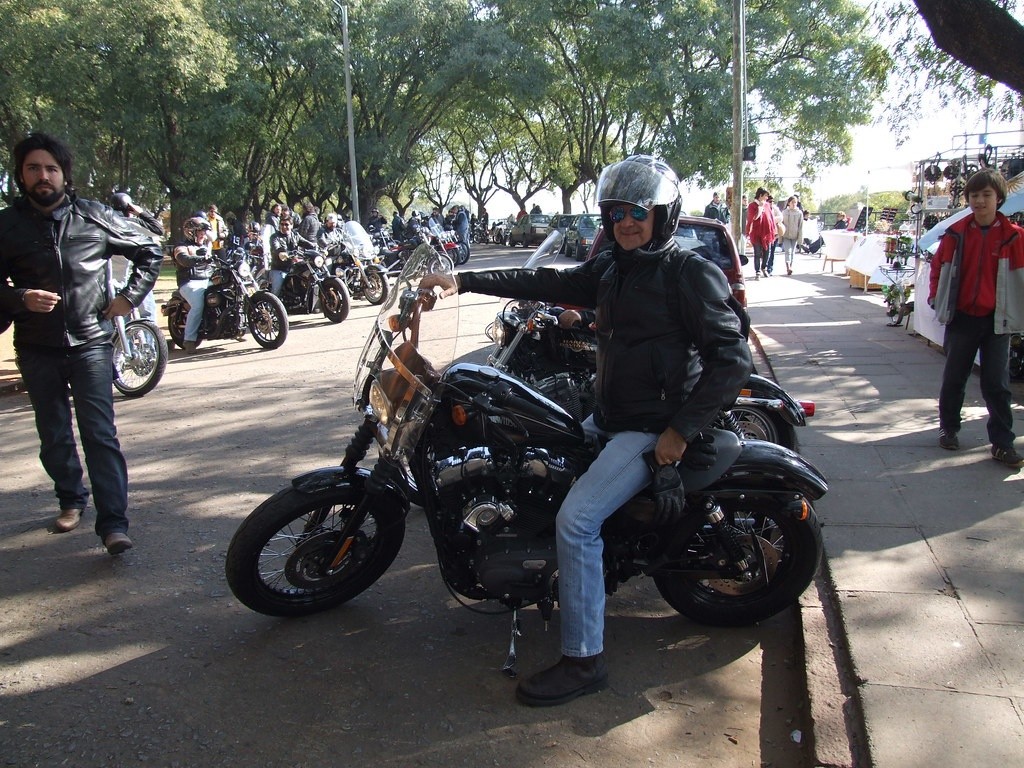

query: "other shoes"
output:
763 268 770 278
182 339 198 354
755 271 762 281
514 653 609 707
787 268 793 276
802 245 810 254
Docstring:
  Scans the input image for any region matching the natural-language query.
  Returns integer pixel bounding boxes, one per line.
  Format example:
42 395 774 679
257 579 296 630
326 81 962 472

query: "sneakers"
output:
990 442 1024 470
939 427 961 451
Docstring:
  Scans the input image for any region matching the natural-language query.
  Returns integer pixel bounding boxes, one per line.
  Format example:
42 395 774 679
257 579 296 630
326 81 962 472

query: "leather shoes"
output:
104 530 134 556
56 506 85 530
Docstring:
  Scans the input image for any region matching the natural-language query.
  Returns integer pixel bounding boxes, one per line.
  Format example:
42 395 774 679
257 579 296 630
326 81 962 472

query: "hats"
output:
326 212 338 224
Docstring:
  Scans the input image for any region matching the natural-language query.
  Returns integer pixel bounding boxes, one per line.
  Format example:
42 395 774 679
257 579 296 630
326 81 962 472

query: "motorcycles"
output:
249 219 513 325
475 227 817 551
160 240 289 351
222 233 831 685
103 253 170 399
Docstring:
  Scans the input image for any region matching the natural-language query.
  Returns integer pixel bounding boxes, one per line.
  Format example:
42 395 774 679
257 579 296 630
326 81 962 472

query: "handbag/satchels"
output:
777 221 786 238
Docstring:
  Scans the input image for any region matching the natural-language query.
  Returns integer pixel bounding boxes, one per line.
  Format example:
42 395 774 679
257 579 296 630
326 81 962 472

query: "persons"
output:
704 187 810 279
418 153 752 708
172 201 374 356
368 205 487 247
101 193 163 357
927 168 1024 469
517 206 527 221
801 211 848 253
530 203 542 214
0 134 164 553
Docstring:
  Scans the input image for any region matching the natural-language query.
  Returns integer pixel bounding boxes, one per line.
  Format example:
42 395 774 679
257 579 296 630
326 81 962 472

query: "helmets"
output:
183 217 212 245
371 208 380 213
596 153 683 242
108 192 132 211
193 211 207 220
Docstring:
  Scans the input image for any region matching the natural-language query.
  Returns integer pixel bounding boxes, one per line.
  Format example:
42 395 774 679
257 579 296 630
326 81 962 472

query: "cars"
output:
544 214 577 254
564 214 604 262
508 214 556 249
586 215 749 308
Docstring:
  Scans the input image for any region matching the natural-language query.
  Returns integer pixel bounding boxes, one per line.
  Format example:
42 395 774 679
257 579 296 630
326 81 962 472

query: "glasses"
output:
605 203 650 223
767 200 775 203
279 221 291 227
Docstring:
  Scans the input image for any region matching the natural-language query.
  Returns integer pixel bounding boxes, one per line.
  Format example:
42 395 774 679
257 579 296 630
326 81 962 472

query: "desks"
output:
879 264 914 326
846 235 918 294
820 230 860 273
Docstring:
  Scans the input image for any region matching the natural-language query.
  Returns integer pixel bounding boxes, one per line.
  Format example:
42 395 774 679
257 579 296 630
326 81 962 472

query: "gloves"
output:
641 446 686 522
680 435 718 472
